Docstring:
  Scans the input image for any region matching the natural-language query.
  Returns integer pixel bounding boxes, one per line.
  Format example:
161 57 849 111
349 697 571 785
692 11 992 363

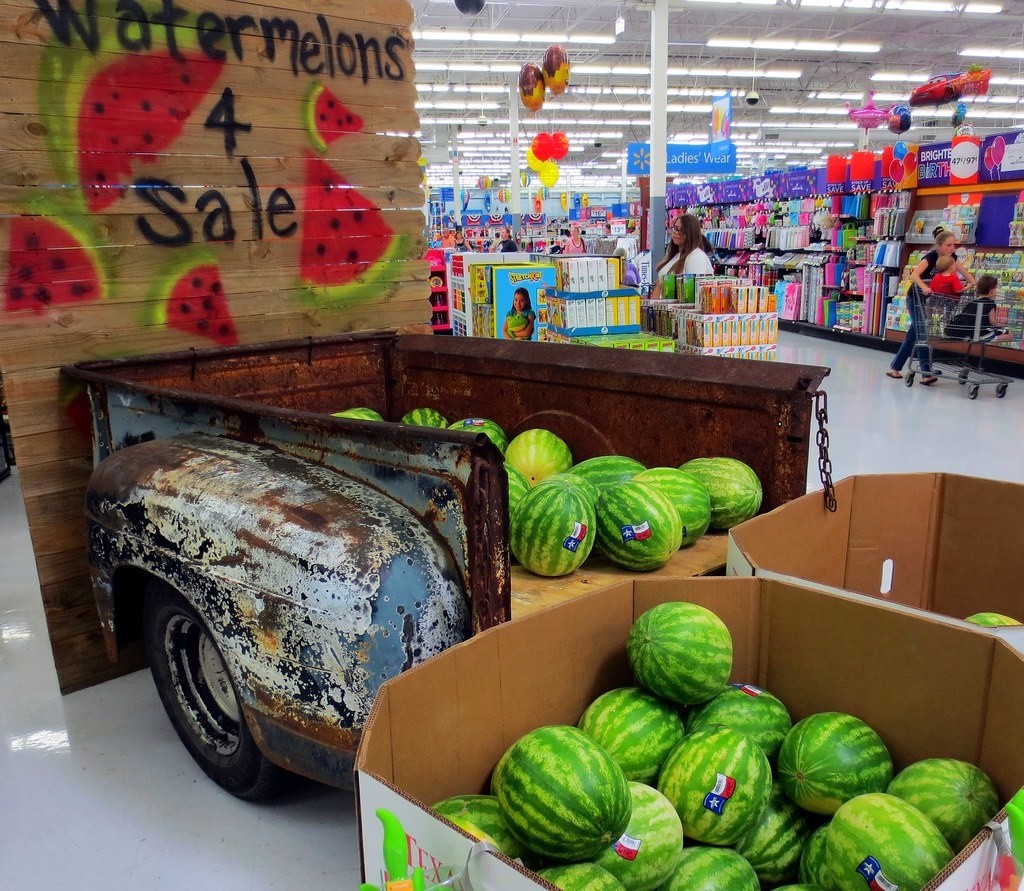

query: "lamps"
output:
409 0 1023 192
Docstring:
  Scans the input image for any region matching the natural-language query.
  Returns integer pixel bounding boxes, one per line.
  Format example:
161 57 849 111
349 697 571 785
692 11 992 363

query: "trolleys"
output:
903 277 1015 400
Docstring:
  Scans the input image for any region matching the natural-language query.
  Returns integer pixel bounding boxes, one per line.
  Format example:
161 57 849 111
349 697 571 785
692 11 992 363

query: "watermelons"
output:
963 613 1022 627
426 601 998 891
328 408 763 578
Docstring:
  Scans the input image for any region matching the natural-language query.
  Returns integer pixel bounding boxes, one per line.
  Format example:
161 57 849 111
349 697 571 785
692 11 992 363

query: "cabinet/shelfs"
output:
662 185 913 319
428 204 641 254
426 249 453 334
884 179 1024 363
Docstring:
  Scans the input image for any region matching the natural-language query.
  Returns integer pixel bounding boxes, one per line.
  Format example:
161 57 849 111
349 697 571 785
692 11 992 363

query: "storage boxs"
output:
726 472 1024 654
471 252 778 361
354 576 1024 891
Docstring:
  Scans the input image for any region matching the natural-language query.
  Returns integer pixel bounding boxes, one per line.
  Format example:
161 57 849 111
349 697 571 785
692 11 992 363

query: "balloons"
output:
542 43 571 97
838 54 995 147
519 62 546 115
499 188 511 202
519 172 531 187
538 187 552 201
477 176 491 190
523 131 578 191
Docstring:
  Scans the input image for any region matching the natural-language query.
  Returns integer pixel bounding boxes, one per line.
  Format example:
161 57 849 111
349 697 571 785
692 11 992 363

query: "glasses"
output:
671 226 685 233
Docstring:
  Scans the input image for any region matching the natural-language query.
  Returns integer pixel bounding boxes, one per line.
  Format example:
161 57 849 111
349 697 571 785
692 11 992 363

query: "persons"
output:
650 213 715 299
565 227 586 254
613 248 641 285
943 274 1014 342
503 287 536 341
925 255 974 326
489 227 518 252
604 224 611 234
427 227 474 252
885 226 978 384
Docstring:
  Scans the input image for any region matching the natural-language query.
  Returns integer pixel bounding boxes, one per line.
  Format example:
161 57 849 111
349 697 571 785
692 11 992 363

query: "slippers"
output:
920 377 937 385
886 372 902 378
